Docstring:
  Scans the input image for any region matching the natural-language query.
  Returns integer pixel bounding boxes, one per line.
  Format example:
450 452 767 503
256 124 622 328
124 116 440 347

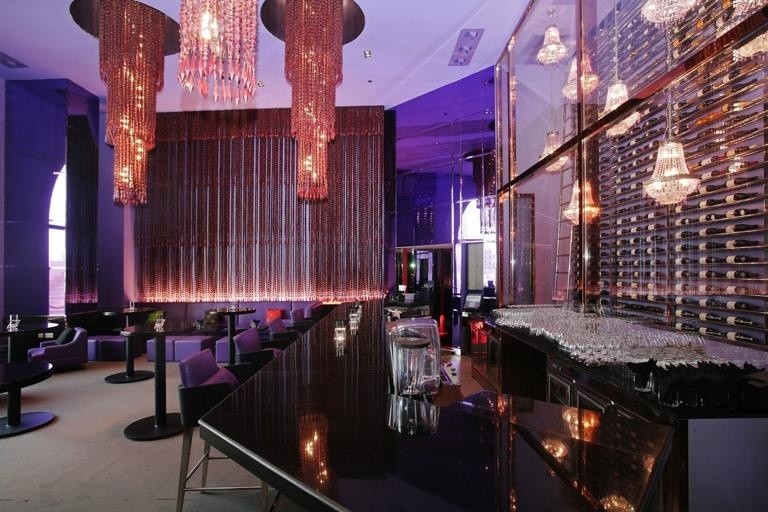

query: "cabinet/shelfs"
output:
468 318 655 425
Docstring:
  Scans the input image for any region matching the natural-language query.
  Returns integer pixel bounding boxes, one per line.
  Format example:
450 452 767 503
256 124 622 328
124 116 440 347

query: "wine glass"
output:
491 303 705 367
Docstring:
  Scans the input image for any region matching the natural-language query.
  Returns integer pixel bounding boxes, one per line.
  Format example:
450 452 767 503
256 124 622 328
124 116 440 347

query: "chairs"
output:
174 327 284 511
27 326 88 373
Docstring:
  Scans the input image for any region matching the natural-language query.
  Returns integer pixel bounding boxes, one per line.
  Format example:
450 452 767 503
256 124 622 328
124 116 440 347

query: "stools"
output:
86 333 238 363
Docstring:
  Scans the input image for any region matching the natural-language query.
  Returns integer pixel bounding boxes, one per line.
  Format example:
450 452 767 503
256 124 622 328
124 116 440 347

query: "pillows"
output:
54 327 76 345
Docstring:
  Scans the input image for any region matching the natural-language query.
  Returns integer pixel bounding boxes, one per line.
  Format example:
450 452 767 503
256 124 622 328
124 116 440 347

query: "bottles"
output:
395 337 433 396
569 1 665 75
674 205 761 226
669 0 761 64
674 255 760 262
684 127 759 157
670 283 760 295
681 111 760 145
674 187 760 214
674 321 754 341
674 296 759 311
673 222 762 239
678 78 762 120
674 309 760 326
672 58 762 111
586 86 669 321
570 149 582 294
579 412 675 512
673 270 761 278
688 144 759 171
687 167 761 198
679 96 760 133
671 239 761 251
690 160 760 180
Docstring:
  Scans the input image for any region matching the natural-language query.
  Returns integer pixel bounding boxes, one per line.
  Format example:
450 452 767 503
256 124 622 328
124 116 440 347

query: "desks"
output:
101 307 155 384
120 320 201 441
0 362 54 393
0 322 60 438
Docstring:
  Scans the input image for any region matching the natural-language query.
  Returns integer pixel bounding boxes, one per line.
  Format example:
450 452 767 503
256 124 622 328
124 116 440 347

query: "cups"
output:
335 341 347 358
334 304 363 341
129 301 134 311
155 312 163 328
632 373 751 411
9 314 18 328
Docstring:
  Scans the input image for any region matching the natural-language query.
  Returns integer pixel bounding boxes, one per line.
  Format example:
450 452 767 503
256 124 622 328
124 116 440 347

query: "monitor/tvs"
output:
463 289 483 312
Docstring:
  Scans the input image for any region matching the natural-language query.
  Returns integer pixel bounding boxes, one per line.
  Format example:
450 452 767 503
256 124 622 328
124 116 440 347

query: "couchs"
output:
112 300 322 339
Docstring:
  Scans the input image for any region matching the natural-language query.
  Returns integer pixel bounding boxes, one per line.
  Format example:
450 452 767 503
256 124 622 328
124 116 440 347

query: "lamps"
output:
69 1 174 212
174 0 265 108
271 1 351 206
532 0 704 226
